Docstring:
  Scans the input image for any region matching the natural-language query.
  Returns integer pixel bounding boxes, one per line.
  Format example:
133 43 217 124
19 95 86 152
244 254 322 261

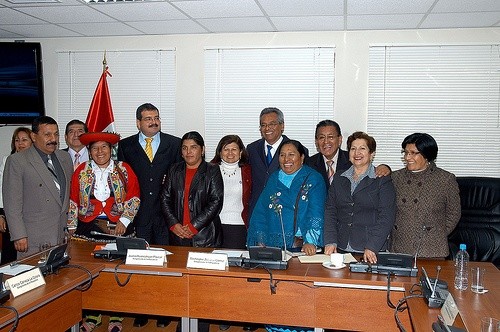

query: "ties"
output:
47 154 59 195
144 139 153 158
266 144 272 165
325 160 333 183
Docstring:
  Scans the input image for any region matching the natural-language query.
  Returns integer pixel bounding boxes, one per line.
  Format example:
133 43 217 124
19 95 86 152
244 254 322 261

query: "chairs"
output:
447 176 500 268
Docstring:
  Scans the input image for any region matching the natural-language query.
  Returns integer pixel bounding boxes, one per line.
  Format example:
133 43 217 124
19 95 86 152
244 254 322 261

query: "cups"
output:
331 253 345 267
480 317 500 332
470 267 485 292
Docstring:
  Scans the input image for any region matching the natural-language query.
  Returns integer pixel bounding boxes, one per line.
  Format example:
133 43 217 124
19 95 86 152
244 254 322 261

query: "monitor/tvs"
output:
0 42 46 126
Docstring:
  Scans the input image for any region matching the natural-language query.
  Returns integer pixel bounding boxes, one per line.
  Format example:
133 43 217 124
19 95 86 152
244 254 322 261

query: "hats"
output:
78 131 122 143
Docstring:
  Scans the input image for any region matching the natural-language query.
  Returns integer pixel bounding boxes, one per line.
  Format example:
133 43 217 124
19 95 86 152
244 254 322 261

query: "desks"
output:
0 241 500 332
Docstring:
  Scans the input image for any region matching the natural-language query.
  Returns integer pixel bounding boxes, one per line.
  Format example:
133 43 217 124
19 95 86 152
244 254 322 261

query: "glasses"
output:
400 149 422 155
138 116 162 121
348 144 369 151
316 134 338 140
259 123 278 128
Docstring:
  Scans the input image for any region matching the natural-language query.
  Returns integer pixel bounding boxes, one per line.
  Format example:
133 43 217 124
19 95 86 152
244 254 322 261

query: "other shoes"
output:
219 324 230 330
156 317 172 328
79 315 102 332
243 325 256 331
133 316 148 327
108 317 122 332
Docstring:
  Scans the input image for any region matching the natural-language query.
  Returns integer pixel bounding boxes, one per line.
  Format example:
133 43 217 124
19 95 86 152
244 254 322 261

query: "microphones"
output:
90 231 150 248
9 244 59 267
431 265 441 298
413 225 426 269
278 207 287 251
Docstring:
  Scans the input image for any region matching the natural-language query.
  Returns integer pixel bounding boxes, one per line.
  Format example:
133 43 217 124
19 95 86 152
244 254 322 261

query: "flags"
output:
84 71 119 167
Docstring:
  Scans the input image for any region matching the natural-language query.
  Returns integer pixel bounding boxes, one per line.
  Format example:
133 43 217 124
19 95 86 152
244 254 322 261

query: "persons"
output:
160 131 224 332
0 127 34 266
209 135 257 330
60 119 93 172
118 102 184 328
3 116 74 260
304 120 391 194
67 131 140 332
387 133 461 258
246 139 331 332
247 106 309 215
323 132 395 264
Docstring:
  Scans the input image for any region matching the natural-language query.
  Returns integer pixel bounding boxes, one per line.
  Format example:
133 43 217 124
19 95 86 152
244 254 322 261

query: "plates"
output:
322 262 346 269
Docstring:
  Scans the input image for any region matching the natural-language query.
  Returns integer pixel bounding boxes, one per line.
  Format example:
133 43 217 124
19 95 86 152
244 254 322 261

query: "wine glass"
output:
39 242 51 263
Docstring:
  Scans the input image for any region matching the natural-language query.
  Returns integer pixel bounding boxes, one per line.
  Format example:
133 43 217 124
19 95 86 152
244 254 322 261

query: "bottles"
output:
453 244 469 290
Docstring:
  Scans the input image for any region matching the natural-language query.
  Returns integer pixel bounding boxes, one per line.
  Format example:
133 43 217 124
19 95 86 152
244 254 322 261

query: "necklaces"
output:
97 163 110 181
